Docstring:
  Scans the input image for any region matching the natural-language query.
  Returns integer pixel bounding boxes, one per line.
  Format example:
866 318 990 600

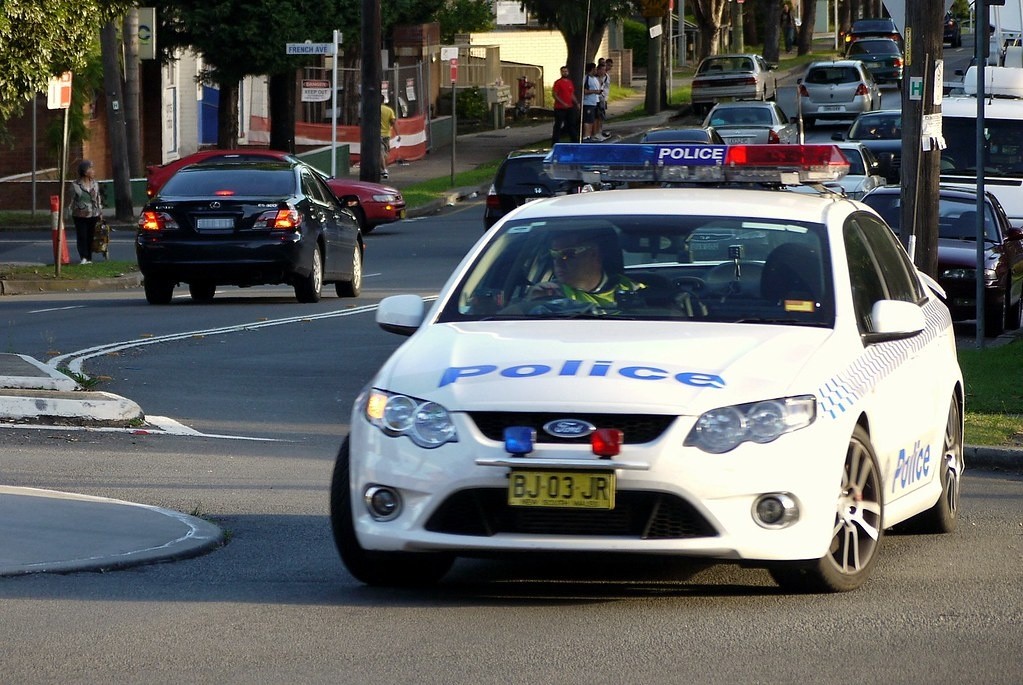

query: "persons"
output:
582 63 604 143
780 3 796 55
63 160 104 265
522 225 649 316
380 94 401 176
551 66 581 148
594 58 614 140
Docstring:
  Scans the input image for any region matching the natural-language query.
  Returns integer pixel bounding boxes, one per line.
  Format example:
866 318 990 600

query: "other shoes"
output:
583 137 594 143
86 261 92 264
383 171 389 178
81 257 87 265
596 135 607 141
602 132 611 138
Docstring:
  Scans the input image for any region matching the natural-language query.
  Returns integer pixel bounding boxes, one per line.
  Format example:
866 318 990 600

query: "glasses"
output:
607 64 613 67
548 245 589 263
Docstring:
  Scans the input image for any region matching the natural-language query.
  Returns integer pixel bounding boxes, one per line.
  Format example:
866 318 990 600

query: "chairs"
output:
815 71 826 79
742 63 751 69
761 243 821 299
959 211 987 236
711 65 723 71
589 226 624 276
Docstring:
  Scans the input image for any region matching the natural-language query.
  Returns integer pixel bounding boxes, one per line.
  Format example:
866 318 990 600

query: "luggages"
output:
94 217 110 260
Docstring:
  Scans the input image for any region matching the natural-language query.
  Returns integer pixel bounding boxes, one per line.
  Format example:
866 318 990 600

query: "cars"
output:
690 52 778 114
943 10 962 48
134 147 407 304
797 60 882 130
831 109 902 183
331 141 964 592
483 125 886 238
700 100 800 145
840 18 904 90
859 183 1023 337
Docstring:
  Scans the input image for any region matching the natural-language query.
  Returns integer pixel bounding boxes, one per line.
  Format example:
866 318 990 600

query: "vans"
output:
936 66 1023 228
